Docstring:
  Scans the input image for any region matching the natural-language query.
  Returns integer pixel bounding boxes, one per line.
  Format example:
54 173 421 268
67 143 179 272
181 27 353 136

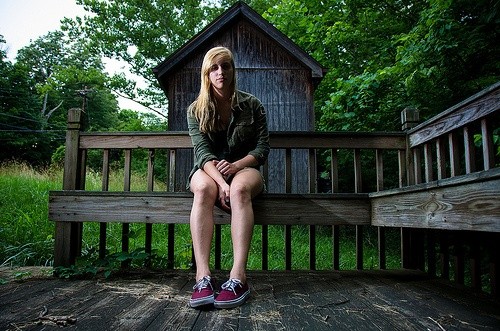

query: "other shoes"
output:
213 278 251 308
189 276 217 307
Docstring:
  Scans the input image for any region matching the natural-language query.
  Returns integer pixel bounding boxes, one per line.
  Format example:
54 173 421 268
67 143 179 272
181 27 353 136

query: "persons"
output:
184 46 272 308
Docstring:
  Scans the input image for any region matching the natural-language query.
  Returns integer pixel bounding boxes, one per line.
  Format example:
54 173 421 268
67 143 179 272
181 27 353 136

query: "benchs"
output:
48 81 500 299
